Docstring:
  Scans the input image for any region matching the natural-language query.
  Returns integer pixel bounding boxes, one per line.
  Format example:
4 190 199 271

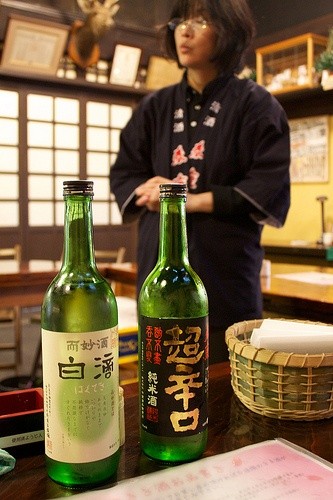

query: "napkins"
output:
251 319 333 354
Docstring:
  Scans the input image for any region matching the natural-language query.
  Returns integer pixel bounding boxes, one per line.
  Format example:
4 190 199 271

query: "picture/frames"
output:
1 19 68 77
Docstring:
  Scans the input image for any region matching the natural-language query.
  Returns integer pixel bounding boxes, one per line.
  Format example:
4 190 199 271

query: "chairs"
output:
30 245 126 380
0 244 23 378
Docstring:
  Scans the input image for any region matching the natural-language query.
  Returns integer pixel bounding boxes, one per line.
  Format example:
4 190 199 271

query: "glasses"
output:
168 18 211 31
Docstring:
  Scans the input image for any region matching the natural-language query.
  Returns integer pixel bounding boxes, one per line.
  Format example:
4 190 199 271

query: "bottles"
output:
138 183 209 461
41 181 120 487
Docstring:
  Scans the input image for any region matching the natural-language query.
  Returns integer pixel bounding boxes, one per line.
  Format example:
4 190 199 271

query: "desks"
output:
0 241 333 500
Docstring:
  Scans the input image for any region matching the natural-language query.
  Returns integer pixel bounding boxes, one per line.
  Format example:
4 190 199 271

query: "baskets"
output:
224 318 333 421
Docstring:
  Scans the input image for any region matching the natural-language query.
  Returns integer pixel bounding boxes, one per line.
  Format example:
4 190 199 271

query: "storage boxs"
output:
0 387 44 455
255 33 333 95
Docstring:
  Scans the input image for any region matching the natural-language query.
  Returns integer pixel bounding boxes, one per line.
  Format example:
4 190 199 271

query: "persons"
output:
110 0 291 364
